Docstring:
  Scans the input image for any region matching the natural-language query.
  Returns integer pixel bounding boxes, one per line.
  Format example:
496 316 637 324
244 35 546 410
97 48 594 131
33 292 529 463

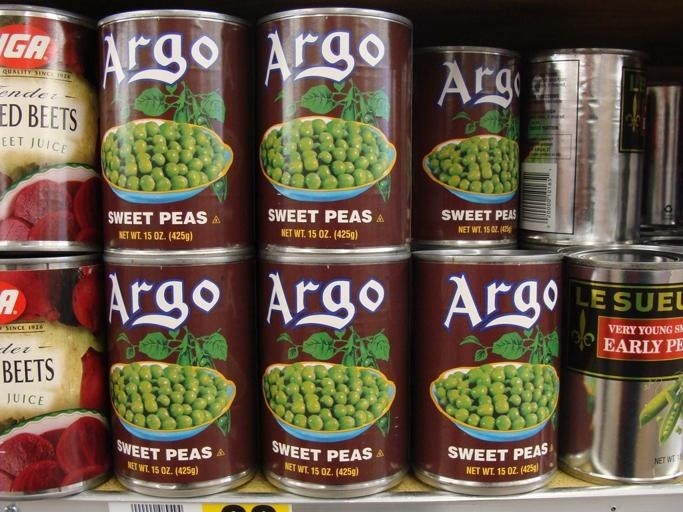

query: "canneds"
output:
413 249 561 496
253 7 413 255
98 9 252 259
0 254 114 502
412 48 520 247
102 251 258 497
255 250 410 499
561 249 683 484
520 47 682 246
0 4 98 252
